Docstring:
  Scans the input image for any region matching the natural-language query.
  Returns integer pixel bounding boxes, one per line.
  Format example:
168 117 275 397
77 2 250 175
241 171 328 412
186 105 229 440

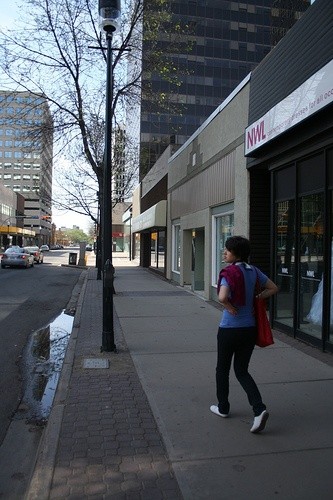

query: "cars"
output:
85 245 93 251
0 246 35 270
24 246 44 264
39 245 50 253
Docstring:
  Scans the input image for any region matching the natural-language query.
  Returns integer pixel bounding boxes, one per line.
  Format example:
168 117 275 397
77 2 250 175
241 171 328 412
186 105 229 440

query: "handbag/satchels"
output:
253 266 274 348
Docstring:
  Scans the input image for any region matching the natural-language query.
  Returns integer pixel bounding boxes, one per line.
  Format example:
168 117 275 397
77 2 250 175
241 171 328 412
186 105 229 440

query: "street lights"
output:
30 224 33 246
7 221 10 248
39 226 43 248
87 0 133 355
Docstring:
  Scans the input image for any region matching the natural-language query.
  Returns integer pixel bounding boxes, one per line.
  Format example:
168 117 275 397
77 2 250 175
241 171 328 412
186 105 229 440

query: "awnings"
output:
132 200 166 235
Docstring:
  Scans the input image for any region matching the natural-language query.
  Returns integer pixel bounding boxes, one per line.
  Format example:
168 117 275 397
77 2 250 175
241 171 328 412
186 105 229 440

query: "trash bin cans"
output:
69 253 77 265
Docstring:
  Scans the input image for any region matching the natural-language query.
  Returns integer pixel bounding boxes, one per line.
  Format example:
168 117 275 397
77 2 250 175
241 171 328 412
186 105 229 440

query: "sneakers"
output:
249 410 269 433
210 404 229 418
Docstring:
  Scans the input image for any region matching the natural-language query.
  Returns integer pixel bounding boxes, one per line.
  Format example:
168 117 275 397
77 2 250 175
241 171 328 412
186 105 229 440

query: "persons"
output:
210 236 279 432
